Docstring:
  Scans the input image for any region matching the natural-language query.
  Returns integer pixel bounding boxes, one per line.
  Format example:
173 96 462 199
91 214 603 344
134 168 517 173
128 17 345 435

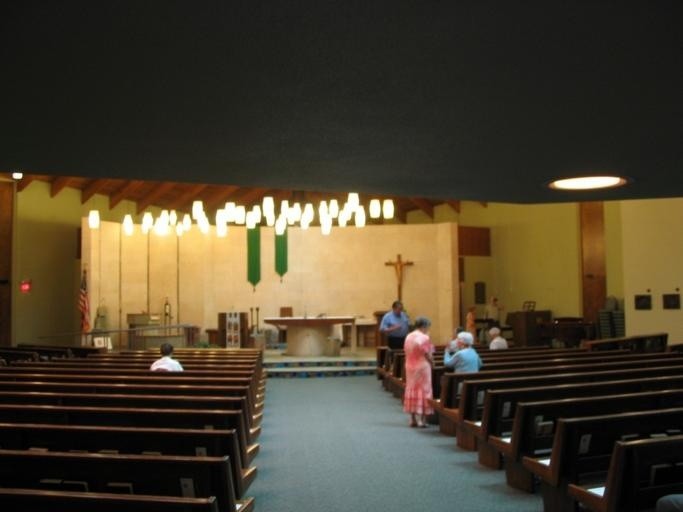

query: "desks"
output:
261 315 355 359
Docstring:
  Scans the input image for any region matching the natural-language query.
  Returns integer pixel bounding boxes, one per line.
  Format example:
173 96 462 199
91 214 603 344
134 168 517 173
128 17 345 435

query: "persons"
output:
442 331 484 372
488 328 508 350
404 316 435 427
444 327 466 352
377 301 411 348
485 296 505 325
149 343 184 374
466 305 482 346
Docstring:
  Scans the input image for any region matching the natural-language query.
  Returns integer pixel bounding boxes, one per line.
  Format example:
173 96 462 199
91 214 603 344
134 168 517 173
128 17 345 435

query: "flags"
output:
75 267 91 332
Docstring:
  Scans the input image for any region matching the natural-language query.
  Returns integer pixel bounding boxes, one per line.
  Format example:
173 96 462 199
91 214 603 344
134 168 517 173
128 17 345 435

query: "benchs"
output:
0 342 267 511
377 331 683 434
479 389 683 512
